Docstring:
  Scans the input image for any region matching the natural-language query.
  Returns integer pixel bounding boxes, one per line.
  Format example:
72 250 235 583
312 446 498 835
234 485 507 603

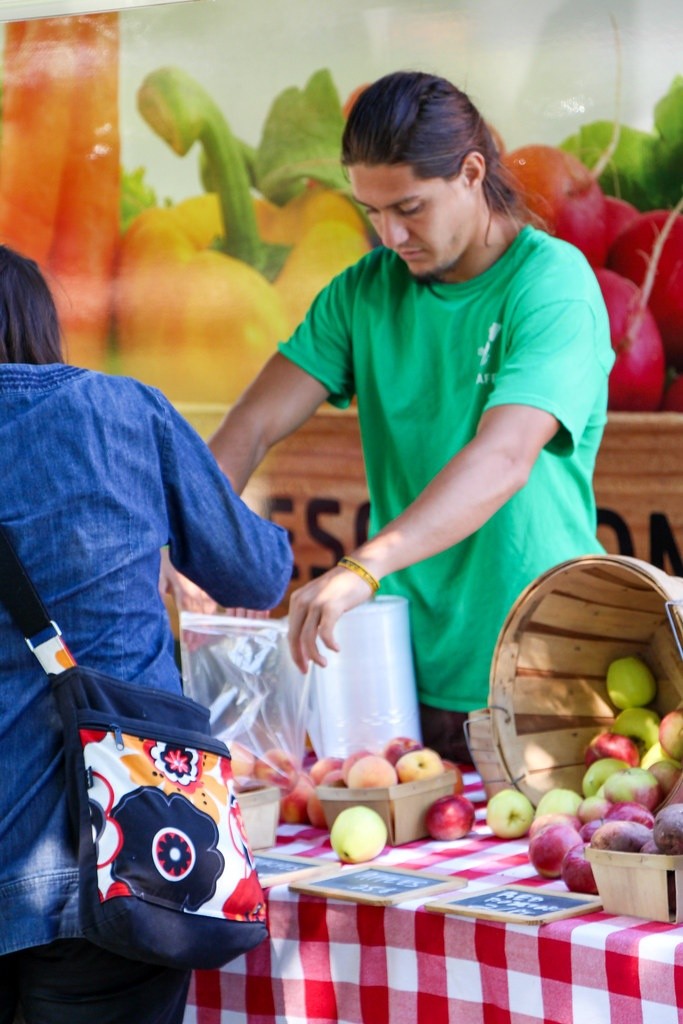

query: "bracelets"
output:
337 553 387 595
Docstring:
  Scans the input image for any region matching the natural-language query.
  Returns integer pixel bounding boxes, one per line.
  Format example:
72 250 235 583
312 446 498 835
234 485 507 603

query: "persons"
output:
162 71 612 763
0 244 291 1024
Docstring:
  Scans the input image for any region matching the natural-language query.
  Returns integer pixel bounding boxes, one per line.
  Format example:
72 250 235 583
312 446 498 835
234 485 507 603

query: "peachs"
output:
584 732 638 767
528 803 682 894
229 738 476 842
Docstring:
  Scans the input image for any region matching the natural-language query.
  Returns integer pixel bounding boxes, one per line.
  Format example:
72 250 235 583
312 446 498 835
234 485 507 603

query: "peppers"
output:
114 65 378 410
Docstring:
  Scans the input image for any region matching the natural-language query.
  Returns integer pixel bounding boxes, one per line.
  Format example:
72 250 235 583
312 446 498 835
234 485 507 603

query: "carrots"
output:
1 12 124 364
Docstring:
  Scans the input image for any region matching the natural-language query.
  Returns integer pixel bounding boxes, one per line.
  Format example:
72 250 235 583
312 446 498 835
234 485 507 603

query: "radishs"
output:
506 13 683 417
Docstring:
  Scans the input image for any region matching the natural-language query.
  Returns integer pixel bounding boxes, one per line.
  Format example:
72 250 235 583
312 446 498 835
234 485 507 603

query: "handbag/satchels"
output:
48 660 275 962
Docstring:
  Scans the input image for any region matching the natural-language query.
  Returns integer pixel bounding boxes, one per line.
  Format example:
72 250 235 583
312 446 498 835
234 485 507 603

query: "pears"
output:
607 658 683 757
484 760 681 839
331 807 388 863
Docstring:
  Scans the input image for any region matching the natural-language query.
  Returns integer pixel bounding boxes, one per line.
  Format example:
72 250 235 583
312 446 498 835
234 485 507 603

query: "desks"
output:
178 760 683 1022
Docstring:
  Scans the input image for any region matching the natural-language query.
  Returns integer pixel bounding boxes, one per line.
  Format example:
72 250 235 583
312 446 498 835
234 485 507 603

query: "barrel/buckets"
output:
462 555 683 820
